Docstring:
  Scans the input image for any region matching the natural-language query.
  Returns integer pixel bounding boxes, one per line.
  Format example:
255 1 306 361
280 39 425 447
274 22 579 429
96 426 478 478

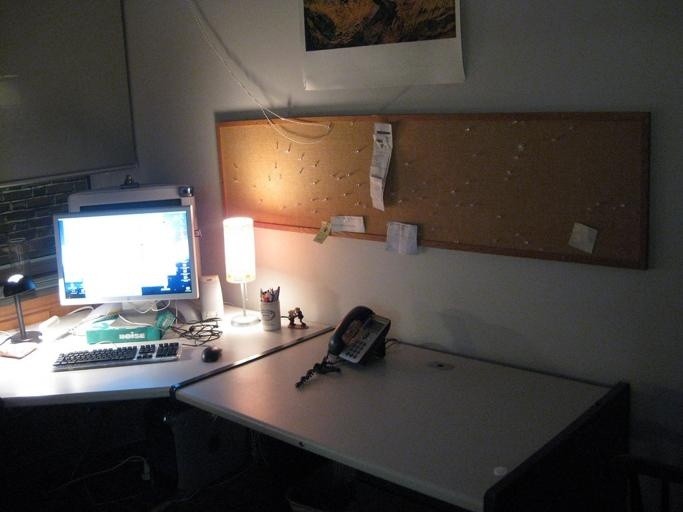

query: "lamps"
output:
222 214 261 327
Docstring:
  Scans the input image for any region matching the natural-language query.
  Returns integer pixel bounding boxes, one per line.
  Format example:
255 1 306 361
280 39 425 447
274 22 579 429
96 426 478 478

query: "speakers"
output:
200 274 224 322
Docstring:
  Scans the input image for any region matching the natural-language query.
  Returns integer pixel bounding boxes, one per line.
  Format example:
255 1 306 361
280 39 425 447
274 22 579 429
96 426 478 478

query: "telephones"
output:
328 306 391 365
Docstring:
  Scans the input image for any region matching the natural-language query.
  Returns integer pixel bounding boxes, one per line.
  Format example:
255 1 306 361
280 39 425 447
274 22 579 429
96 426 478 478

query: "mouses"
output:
202 346 222 362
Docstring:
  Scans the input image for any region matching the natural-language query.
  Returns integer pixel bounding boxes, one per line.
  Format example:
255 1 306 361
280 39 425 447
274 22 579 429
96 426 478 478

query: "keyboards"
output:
52 340 182 370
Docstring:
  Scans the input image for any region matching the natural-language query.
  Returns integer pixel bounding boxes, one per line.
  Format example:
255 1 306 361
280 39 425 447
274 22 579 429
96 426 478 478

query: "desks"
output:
173 329 626 511
0 305 333 408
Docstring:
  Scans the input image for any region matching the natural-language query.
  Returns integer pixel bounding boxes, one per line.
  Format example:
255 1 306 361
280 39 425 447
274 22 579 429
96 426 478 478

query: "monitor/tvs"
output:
67 183 203 324
53 203 199 328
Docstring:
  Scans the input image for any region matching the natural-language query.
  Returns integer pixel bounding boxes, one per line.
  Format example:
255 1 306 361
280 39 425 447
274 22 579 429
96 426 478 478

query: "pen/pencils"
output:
260 287 280 302
